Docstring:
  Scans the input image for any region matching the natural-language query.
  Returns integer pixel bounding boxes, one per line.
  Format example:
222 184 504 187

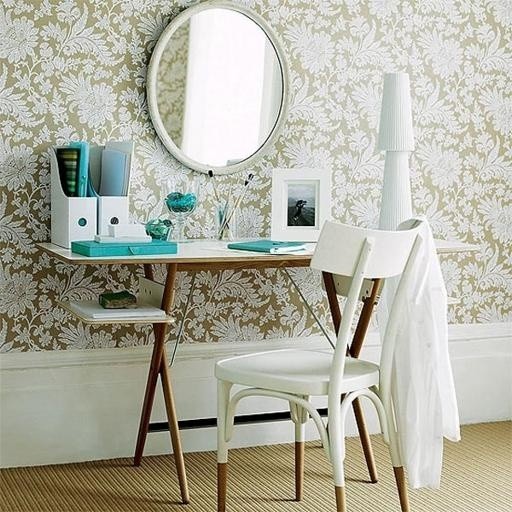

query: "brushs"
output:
208 170 255 240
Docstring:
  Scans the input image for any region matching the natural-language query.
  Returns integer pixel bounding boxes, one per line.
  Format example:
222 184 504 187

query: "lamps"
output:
377 72 417 231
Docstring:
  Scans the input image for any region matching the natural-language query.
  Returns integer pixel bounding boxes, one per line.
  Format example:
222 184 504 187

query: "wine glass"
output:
164 200 197 243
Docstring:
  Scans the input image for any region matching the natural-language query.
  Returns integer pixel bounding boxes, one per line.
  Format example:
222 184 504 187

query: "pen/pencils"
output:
209 170 255 240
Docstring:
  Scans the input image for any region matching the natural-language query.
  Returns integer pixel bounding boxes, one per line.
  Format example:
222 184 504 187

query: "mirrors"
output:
144 1 290 176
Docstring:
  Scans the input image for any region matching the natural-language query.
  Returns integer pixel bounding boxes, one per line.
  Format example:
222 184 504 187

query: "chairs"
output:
212 220 431 512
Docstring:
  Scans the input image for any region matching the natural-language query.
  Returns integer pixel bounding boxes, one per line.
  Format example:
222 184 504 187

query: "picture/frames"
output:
271 168 331 244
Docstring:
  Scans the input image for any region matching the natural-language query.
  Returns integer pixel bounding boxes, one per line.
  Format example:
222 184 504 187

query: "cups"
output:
144 224 174 241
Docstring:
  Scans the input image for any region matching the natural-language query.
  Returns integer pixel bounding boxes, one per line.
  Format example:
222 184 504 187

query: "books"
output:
227 239 306 255
99 290 137 309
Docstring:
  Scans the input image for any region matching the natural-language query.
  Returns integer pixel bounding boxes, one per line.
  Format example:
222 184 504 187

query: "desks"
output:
35 239 378 503
